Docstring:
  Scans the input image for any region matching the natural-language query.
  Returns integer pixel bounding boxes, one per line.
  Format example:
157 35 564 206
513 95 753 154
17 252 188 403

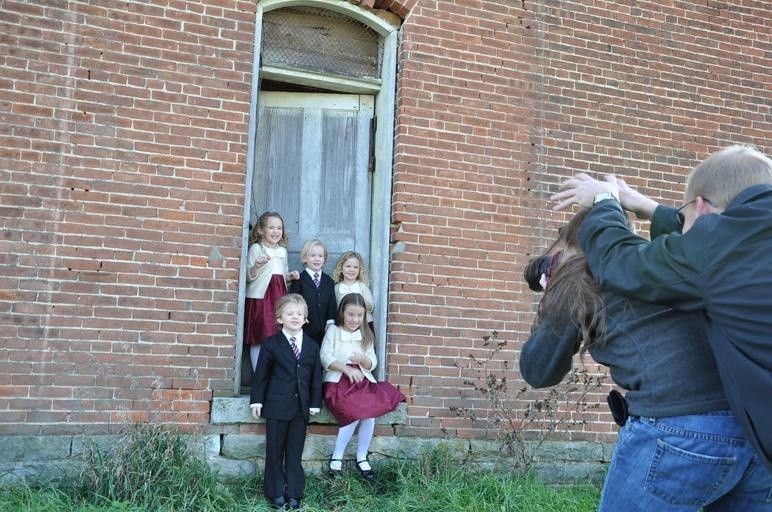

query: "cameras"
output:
523 250 561 294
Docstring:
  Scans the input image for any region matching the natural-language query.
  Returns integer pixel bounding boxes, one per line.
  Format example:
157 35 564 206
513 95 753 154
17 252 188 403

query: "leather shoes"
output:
289 495 299 510
271 495 285 509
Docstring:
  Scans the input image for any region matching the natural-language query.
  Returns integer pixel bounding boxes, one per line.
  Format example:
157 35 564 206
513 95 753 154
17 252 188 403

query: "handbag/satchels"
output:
604 390 629 426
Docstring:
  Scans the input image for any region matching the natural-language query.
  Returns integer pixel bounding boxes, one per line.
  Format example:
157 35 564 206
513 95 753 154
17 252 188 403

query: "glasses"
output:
672 196 713 228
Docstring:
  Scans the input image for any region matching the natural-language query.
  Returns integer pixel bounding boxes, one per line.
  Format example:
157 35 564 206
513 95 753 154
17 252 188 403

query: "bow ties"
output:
338 283 359 295
340 329 362 342
265 247 285 258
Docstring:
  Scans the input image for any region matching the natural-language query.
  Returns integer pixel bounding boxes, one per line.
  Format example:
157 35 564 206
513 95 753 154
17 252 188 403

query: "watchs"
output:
592 192 619 206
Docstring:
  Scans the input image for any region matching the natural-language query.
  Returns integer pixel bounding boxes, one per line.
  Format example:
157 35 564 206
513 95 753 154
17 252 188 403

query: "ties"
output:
313 272 320 287
290 337 300 360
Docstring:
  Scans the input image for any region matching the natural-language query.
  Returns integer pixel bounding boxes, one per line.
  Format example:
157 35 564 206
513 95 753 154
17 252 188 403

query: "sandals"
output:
356 458 375 478
328 454 343 477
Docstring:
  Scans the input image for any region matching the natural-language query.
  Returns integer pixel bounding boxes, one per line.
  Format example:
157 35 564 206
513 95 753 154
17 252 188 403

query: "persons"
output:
244 210 300 373
289 239 338 349
332 251 376 336
320 292 407 480
250 294 323 512
519 207 772 511
550 147 772 477
